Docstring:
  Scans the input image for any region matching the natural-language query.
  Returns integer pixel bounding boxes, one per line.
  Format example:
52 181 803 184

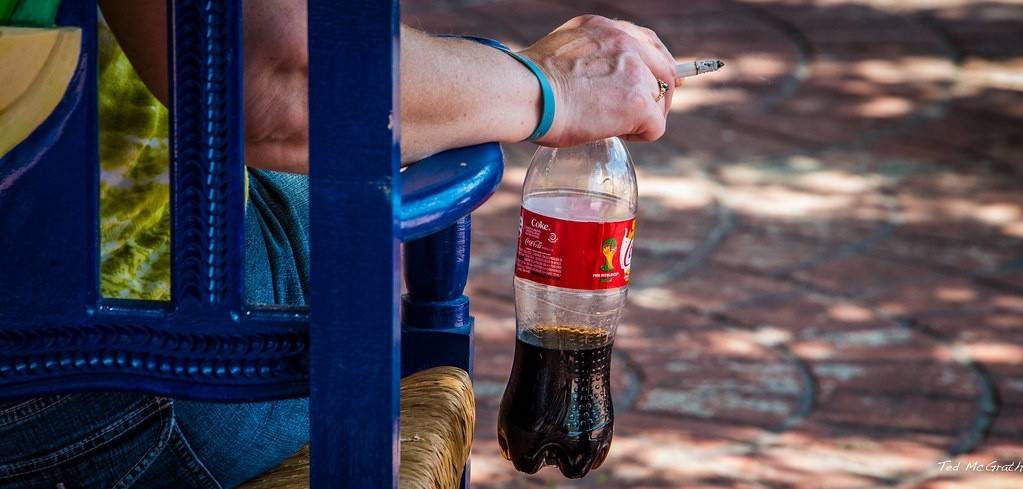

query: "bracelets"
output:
433 31 557 142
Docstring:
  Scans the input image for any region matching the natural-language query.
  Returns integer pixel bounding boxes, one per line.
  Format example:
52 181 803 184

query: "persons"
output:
0 0 684 489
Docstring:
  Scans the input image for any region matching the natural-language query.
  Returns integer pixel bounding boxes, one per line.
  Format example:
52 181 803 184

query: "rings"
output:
654 77 670 104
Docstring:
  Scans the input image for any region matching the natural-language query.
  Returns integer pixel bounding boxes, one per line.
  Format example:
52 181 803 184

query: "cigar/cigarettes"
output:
674 59 725 79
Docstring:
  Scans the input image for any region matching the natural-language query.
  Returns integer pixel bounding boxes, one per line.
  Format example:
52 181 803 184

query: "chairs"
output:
1 0 506 489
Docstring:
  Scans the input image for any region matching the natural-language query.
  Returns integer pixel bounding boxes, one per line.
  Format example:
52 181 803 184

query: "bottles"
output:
497 128 638 481
